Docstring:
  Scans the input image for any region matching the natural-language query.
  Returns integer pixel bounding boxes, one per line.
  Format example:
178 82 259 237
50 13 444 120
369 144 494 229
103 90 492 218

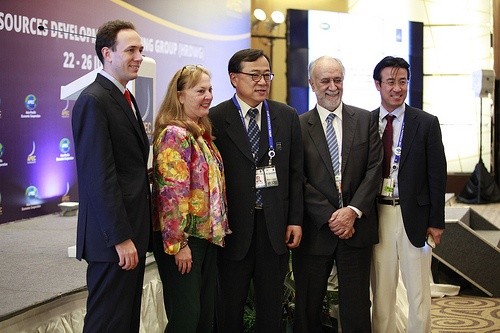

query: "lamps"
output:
263 10 286 35
251 8 267 30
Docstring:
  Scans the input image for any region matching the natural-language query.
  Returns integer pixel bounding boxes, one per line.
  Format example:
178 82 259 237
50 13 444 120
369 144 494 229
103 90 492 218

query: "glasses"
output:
180 64 206 76
381 80 409 87
231 71 274 82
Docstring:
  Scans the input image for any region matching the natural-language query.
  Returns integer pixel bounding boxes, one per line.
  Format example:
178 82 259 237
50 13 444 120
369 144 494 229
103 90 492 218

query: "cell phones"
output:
426 233 436 249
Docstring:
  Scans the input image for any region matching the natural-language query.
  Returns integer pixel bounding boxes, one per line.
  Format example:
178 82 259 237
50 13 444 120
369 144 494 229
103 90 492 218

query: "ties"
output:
247 108 263 209
379 114 396 199
123 90 133 111
326 113 342 210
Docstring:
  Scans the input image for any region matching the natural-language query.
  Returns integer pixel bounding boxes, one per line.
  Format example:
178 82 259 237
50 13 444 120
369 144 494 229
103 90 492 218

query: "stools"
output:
57 201 79 218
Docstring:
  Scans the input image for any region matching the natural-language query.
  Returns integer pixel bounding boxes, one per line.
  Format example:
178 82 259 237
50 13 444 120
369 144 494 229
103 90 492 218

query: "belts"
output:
374 199 400 205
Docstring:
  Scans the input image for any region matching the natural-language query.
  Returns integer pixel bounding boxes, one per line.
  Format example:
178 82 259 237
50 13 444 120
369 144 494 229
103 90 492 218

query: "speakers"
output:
473 70 495 96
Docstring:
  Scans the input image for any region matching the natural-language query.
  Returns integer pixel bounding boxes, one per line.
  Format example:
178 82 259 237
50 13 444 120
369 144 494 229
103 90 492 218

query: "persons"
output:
370 56 448 333
71 21 153 333
207 49 303 333
151 64 233 333
256 176 261 182
291 56 384 333
